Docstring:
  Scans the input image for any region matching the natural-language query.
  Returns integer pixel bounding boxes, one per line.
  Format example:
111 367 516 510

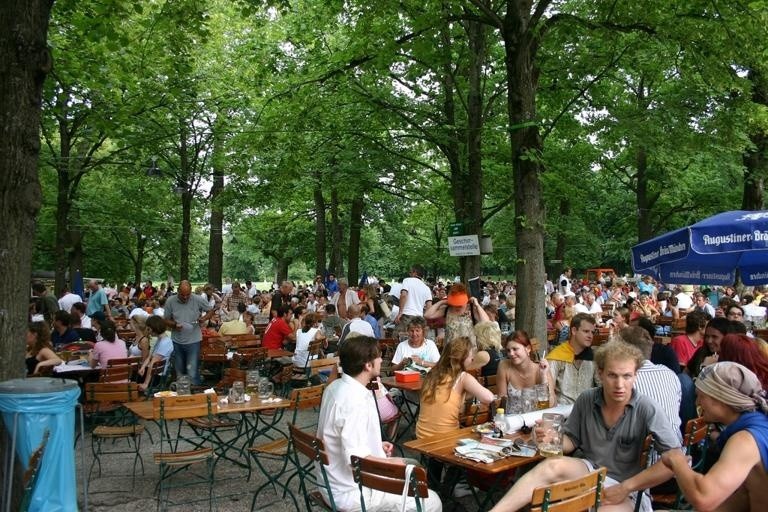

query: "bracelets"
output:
421 358 424 366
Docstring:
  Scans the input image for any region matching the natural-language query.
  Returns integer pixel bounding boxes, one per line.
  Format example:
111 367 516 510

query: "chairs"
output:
36 304 768 512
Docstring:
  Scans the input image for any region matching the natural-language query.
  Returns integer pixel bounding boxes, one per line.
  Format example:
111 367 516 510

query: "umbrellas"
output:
630 209 768 286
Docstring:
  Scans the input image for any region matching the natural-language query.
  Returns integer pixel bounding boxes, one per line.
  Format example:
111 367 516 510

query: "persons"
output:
496 330 556 415
471 319 501 376
620 326 683 464
488 340 682 512
389 314 441 441
477 275 518 334
662 360 768 512
541 265 690 334
425 285 489 346
650 344 695 414
416 336 494 502
393 263 432 340
666 286 768 390
547 314 601 404
27 275 398 381
316 336 444 512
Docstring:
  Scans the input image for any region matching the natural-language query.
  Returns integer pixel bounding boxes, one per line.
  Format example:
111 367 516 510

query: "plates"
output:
474 423 495 433
153 391 177 398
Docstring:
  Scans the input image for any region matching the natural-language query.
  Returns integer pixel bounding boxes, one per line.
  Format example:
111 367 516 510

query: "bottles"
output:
494 407 506 438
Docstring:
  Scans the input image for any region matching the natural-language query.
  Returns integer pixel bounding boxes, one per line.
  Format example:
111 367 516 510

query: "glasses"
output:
502 444 520 454
515 439 537 450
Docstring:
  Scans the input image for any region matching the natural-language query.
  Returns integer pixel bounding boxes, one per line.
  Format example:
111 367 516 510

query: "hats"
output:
564 291 576 298
447 290 468 305
89 312 105 320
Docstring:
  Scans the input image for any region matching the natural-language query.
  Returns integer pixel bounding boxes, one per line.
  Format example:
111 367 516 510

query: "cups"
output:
535 384 550 411
257 377 275 399
169 375 192 396
229 380 245 404
245 369 260 397
539 412 565 458
